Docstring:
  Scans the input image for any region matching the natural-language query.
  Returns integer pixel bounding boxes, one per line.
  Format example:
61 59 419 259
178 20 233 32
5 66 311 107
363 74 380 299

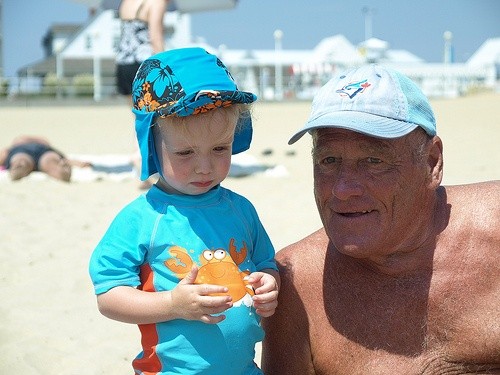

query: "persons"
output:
261 63 500 375
0 135 92 182
116 0 169 107
89 46 281 375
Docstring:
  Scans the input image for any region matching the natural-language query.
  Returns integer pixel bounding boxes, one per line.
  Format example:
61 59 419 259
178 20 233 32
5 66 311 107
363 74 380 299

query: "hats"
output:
287 63 437 145
132 47 257 181
